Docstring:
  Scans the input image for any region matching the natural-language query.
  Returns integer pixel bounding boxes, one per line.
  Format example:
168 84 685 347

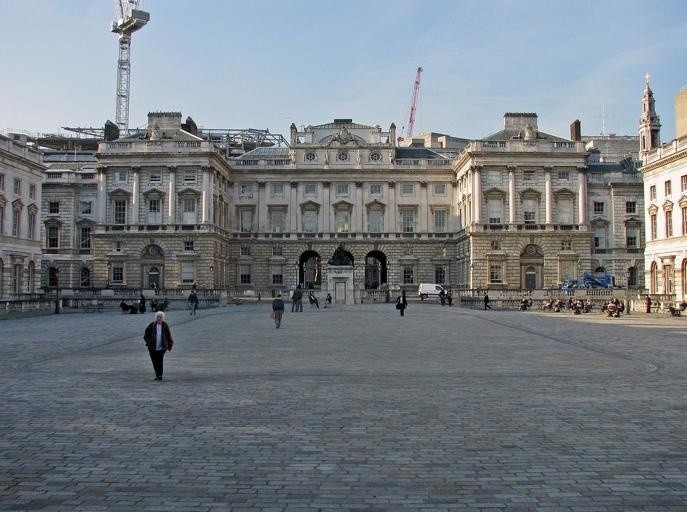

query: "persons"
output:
396 292 408 316
440 288 454 307
483 292 491 311
120 294 169 314
325 293 332 305
518 296 625 317
644 295 650 313
272 294 285 329
187 290 198 315
675 300 686 317
291 287 303 313
309 292 320 308
144 311 173 380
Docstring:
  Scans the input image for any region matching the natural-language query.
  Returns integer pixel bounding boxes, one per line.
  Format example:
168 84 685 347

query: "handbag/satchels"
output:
269 310 275 321
395 303 400 310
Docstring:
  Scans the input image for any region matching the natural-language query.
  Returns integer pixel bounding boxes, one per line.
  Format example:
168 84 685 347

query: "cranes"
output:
108 0 152 130
406 65 424 136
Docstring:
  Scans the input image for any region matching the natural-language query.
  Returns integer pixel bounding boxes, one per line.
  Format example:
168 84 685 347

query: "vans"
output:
417 283 447 299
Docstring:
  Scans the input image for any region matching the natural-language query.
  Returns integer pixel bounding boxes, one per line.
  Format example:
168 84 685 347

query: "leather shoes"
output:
154 376 163 381
